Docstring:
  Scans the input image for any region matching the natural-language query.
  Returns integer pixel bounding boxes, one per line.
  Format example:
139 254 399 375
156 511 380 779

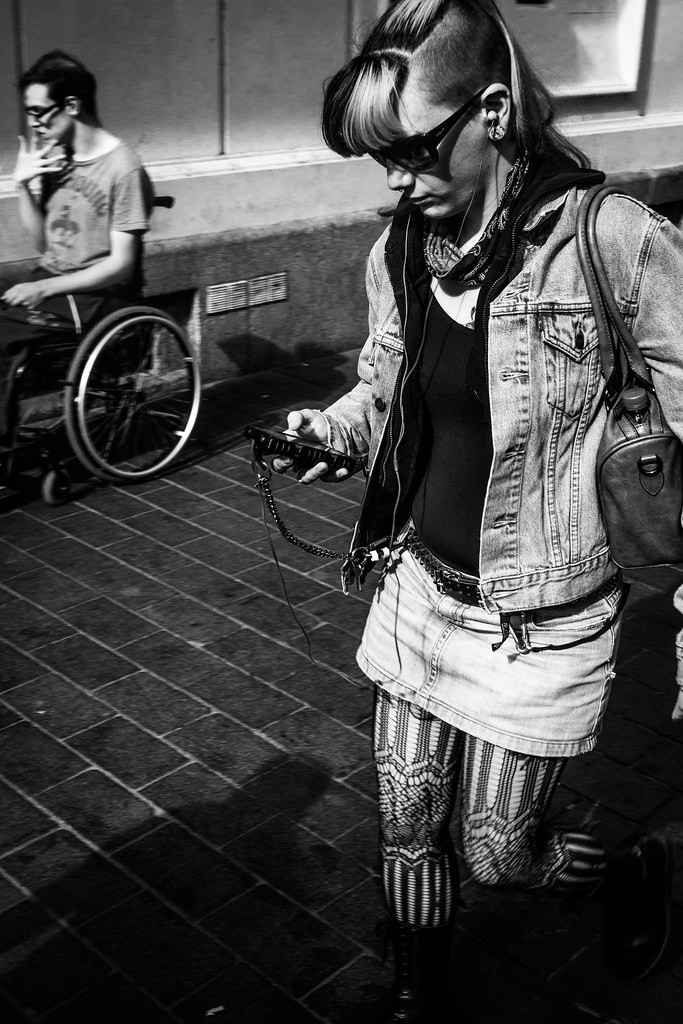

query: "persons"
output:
0 49 154 507
272 1 683 1024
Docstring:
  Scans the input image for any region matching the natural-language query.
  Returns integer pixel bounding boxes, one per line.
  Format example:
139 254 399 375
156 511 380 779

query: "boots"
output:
619 830 674 985
374 914 454 1024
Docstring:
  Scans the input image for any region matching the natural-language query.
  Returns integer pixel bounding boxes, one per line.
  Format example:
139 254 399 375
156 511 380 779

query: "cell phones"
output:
246 422 354 473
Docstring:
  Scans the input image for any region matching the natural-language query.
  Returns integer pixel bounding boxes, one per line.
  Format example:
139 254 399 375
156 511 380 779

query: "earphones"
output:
488 109 500 125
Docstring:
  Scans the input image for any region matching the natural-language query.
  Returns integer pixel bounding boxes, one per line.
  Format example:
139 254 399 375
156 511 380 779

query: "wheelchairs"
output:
0 197 201 505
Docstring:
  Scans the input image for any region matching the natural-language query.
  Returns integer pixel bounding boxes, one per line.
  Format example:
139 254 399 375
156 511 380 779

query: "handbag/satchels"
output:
575 183 683 569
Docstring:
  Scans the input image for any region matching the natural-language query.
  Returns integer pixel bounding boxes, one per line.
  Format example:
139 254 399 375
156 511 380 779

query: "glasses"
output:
23 101 66 118
368 84 492 174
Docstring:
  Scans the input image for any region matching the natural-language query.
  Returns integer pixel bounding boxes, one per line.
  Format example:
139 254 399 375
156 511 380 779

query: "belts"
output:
401 528 481 607
